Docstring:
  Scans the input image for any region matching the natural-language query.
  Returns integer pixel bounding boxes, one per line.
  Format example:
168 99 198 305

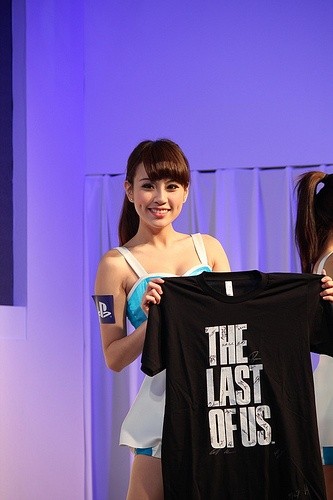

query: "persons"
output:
294 170 333 500
89 137 236 499
